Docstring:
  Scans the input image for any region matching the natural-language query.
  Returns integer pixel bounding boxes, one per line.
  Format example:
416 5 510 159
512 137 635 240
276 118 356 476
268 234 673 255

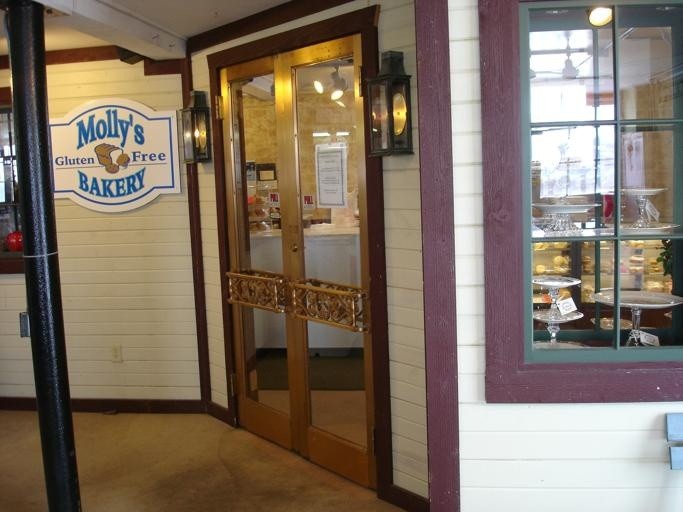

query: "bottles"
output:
623 306 645 346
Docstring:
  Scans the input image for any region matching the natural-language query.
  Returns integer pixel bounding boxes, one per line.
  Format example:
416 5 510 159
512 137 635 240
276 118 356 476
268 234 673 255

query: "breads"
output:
535 239 672 303
95 144 130 173
248 197 279 231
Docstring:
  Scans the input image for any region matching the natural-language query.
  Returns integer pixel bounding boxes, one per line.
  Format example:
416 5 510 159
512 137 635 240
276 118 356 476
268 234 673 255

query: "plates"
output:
589 317 633 331
590 289 683 310
531 202 601 214
622 222 681 236
619 187 669 197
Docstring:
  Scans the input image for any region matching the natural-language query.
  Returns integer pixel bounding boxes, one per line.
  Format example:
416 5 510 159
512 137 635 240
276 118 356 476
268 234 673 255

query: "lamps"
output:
175 89 211 166
312 65 353 108
583 5 613 28
364 51 414 158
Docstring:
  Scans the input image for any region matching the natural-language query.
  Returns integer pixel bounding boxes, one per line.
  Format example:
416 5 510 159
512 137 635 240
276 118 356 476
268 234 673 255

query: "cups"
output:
302 213 313 229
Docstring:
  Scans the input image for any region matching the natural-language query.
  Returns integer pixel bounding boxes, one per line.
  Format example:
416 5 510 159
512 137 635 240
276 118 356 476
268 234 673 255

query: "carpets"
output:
256 348 365 391
